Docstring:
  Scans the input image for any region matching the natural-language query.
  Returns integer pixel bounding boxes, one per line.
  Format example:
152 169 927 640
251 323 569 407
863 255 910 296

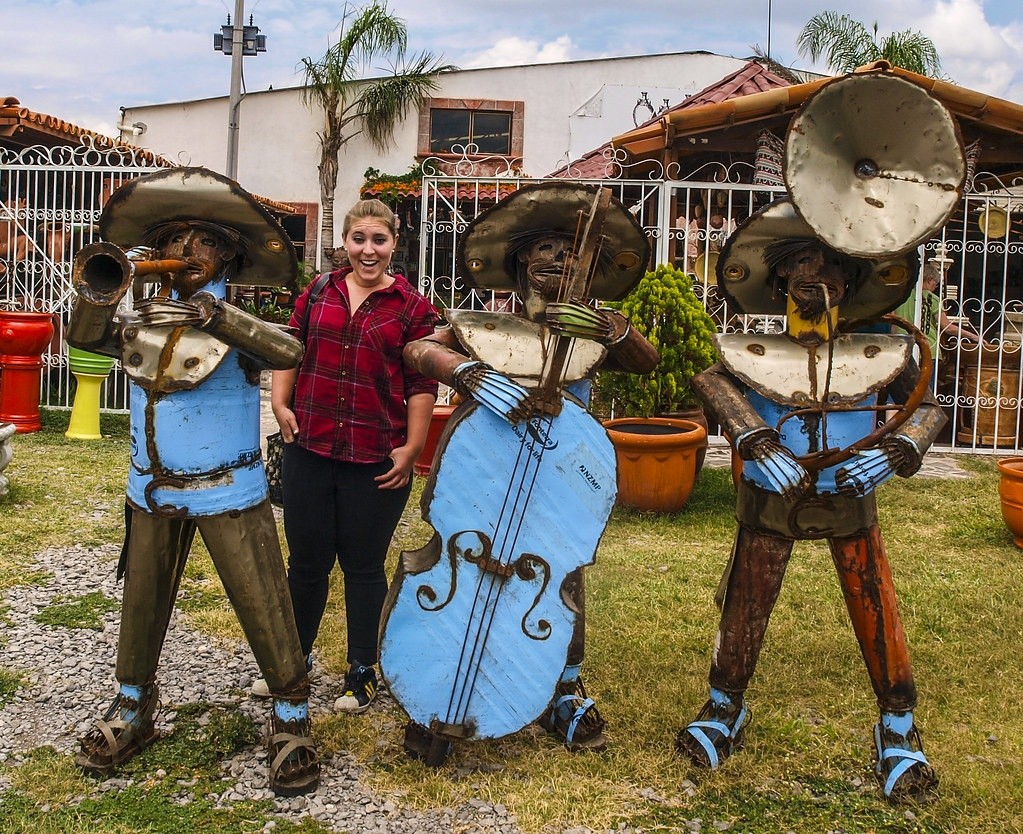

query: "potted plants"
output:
591 262 717 486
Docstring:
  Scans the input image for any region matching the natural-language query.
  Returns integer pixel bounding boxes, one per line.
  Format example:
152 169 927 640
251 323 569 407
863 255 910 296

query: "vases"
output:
601 415 708 513
997 457 1023 550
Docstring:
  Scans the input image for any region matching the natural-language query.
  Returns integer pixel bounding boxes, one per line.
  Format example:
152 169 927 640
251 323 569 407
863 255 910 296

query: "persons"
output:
68 220 322 795
894 265 988 391
252 199 441 712
399 233 663 761
676 239 950 803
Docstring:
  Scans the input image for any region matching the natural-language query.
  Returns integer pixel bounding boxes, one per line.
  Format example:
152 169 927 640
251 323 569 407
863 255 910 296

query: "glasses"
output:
931 278 939 288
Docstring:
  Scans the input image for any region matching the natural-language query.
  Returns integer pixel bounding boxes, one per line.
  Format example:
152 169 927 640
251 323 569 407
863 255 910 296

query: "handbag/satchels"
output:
265 432 283 509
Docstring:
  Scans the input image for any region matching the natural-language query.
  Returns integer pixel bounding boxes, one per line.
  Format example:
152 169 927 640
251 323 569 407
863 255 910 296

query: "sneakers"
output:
251 655 314 697
334 659 378 713
